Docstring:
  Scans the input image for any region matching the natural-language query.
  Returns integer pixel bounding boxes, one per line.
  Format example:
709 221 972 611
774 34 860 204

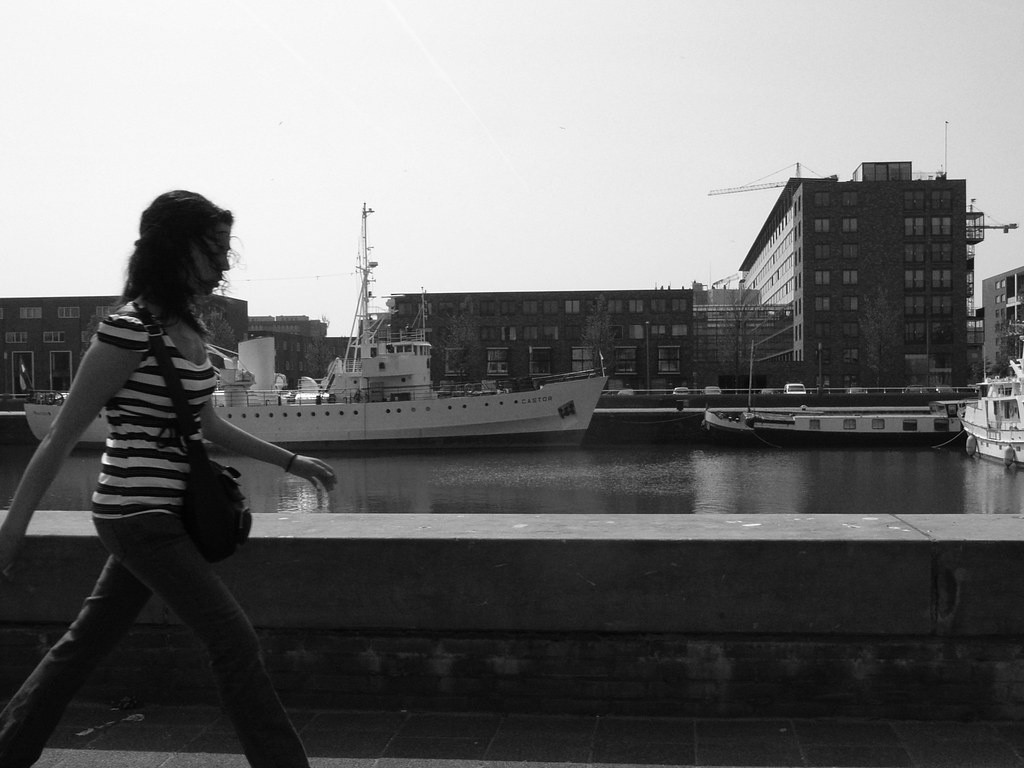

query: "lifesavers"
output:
465 383 475 394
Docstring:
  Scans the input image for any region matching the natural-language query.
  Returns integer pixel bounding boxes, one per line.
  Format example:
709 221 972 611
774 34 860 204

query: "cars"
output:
672 387 691 395
905 385 929 393
702 385 722 396
845 387 869 395
617 389 634 396
930 384 955 393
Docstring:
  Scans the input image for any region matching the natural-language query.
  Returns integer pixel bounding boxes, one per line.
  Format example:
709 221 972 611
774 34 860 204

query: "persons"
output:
0 190 338 768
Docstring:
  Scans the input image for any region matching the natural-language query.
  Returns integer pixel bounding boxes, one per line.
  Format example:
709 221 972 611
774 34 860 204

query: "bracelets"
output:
283 453 298 472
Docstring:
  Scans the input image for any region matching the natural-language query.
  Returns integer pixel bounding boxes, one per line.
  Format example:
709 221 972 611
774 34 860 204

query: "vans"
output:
783 384 807 395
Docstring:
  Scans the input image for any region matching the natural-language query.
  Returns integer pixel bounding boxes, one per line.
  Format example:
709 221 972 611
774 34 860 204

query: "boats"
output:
956 358 1024 469
701 342 969 447
20 202 610 453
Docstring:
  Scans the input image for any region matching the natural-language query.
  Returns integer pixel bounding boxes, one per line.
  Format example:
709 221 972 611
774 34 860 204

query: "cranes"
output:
707 162 839 197
